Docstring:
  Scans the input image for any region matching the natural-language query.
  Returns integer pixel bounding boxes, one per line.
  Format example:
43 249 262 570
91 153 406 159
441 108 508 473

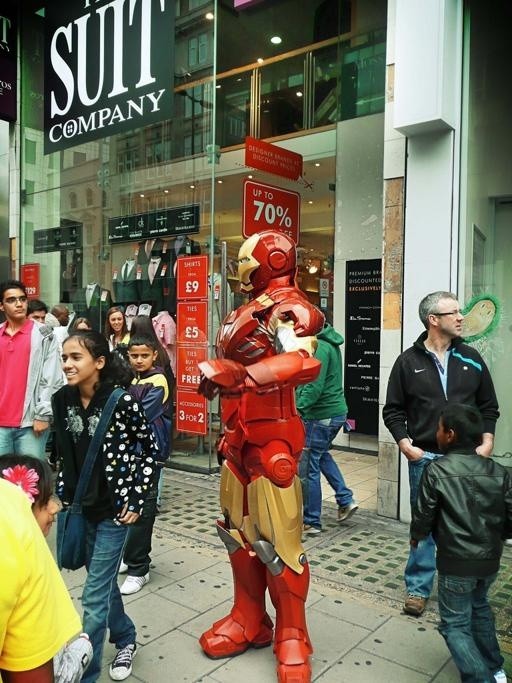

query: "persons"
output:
69 236 200 333
410 402 512 681
291 312 358 537
191 229 328 683
2 281 175 680
383 291 501 618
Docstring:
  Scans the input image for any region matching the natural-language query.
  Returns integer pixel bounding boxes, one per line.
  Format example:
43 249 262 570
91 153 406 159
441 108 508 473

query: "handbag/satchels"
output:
57 502 85 570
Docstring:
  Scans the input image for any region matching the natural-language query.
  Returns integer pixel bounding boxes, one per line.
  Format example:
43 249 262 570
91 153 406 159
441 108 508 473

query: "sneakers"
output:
109 642 137 679
337 500 358 522
302 522 321 533
403 594 426 614
120 571 149 593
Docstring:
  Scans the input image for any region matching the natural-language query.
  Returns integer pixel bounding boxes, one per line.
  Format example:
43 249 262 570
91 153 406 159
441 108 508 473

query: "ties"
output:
124 263 128 280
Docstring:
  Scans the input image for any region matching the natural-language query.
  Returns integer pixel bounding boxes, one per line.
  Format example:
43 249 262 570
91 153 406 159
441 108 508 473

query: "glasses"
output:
433 310 462 315
